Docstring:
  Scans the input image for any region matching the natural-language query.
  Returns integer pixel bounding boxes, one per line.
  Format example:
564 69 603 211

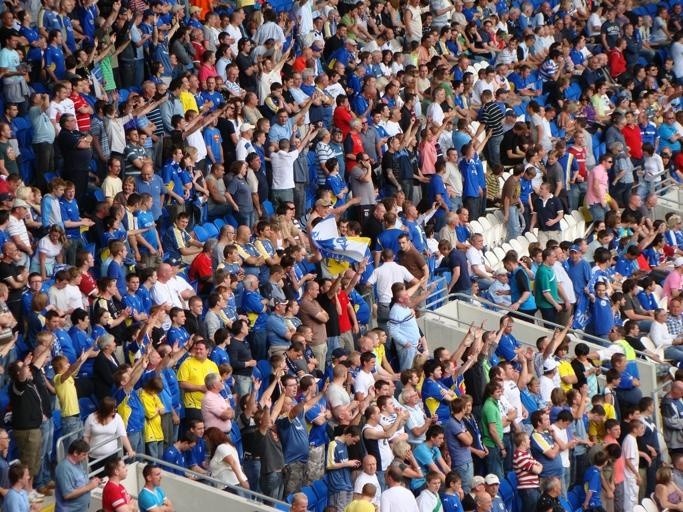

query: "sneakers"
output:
28 482 55 503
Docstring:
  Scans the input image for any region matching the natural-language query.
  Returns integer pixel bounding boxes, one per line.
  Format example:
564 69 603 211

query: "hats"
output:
674 256 683 266
507 109 520 118
316 199 331 207
12 199 30 210
486 474 499 485
347 39 357 46
332 349 349 359
551 49 563 58
543 358 558 372
172 4 201 14
472 476 484 488
310 44 322 52
241 122 255 132
570 245 579 252
497 88 510 95
163 257 182 266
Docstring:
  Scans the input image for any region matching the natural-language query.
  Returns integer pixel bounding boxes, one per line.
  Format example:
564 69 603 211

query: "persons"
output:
1 0 683 512
